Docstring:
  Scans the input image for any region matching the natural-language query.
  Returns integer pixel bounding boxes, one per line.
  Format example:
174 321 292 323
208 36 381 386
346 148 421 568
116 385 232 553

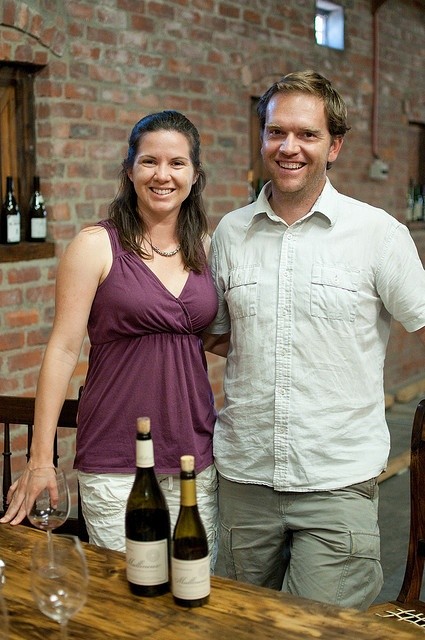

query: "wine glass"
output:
30 534 89 640
25 466 72 578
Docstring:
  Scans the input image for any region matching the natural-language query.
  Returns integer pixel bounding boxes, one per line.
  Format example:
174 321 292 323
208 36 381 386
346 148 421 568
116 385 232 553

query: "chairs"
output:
1 384 88 542
363 396 425 631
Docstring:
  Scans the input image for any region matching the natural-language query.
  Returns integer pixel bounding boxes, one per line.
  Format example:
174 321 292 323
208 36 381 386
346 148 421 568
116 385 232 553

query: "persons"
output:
205 70 424 610
0 110 227 576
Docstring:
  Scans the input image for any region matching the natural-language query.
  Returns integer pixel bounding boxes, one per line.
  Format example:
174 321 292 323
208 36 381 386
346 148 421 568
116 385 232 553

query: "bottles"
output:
170 454 210 608
25 175 47 243
125 416 171 597
256 177 264 199
248 181 256 204
405 183 414 222
413 183 423 221
0 176 21 246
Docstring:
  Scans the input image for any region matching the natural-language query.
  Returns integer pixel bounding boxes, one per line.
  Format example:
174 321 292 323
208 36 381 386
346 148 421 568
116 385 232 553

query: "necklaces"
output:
139 231 182 258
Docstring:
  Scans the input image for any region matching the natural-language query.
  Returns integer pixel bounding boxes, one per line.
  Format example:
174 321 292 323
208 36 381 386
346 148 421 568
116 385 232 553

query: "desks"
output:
0 519 425 640
